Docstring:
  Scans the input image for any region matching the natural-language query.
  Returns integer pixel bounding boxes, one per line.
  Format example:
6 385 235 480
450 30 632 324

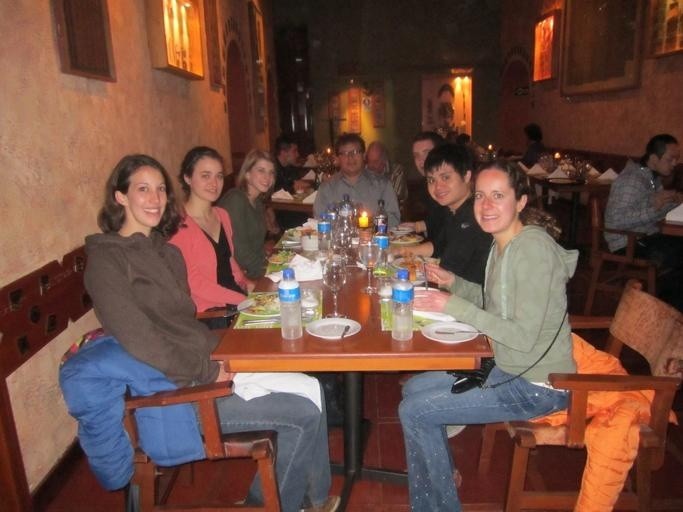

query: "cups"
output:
294 182 303 198
313 158 337 190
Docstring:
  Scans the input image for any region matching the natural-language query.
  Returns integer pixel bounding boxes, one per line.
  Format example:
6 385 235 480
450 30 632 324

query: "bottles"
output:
278 270 302 339
391 270 413 342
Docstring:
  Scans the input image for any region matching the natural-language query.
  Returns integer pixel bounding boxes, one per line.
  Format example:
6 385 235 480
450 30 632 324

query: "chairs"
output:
121 329 281 512
587 195 663 314
478 280 681 512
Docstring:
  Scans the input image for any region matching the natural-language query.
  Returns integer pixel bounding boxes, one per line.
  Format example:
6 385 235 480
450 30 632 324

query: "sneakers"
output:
304 495 339 511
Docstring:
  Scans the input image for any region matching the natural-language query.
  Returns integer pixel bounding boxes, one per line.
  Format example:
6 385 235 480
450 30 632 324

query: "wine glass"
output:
537 151 593 181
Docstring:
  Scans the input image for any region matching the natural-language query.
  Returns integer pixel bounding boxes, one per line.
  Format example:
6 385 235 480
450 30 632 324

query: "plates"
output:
306 318 360 339
419 323 479 345
270 194 438 314
237 294 278 316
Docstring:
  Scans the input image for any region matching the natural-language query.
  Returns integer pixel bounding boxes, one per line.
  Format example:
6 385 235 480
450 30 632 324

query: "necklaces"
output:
641 166 658 192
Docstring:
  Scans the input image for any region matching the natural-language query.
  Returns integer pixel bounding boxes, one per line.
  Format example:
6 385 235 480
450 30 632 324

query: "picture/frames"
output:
529 7 561 85
644 0 683 59
556 0 641 98
51 1 118 84
144 0 205 81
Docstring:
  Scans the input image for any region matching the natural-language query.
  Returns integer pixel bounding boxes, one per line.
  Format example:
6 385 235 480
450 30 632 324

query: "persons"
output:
269 133 323 225
309 132 402 234
163 146 329 443
77 152 345 512
602 133 682 299
215 145 377 431
517 122 546 168
456 134 481 173
394 127 454 236
363 138 408 212
386 138 496 283
395 157 581 511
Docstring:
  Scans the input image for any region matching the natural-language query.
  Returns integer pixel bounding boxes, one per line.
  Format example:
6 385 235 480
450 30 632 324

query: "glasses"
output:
338 149 364 158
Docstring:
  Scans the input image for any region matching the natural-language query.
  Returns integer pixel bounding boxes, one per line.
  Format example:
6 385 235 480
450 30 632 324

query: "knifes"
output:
433 329 485 335
240 314 309 326
340 325 349 338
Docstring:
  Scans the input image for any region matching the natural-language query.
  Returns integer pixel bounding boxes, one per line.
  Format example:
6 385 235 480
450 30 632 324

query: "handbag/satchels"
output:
446 356 496 392
